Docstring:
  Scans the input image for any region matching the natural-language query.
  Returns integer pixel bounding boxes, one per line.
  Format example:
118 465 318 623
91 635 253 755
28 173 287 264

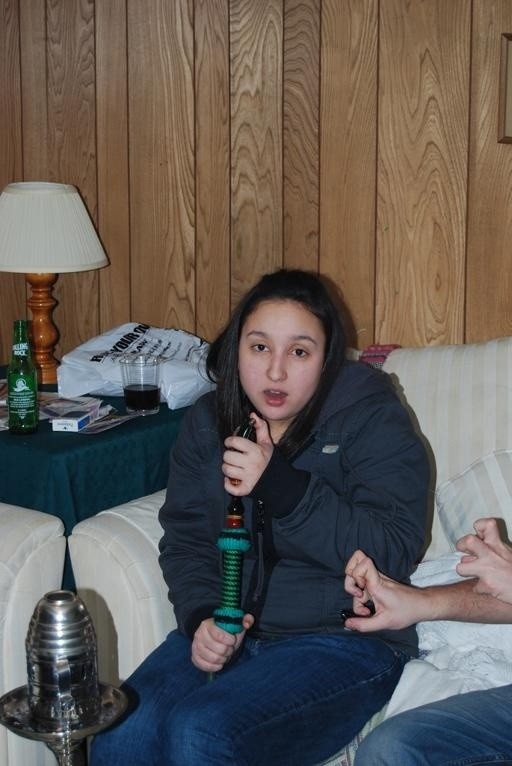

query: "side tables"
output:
1 361 203 524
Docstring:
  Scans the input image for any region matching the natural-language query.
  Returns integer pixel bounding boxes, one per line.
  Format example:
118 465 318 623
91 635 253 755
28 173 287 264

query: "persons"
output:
89 271 432 766
344 517 512 766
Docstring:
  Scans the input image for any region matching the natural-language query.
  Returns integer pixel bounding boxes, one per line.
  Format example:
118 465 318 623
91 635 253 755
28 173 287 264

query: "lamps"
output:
0 182 111 388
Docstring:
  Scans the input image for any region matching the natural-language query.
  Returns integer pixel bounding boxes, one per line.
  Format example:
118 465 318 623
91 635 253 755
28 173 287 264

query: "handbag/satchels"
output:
56 321 220 411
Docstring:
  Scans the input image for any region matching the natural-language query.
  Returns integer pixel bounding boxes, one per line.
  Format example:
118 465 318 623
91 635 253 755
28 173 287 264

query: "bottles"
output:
7 319 38 435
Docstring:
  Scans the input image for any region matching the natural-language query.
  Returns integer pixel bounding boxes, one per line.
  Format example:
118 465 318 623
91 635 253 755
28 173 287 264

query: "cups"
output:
118 355 162 415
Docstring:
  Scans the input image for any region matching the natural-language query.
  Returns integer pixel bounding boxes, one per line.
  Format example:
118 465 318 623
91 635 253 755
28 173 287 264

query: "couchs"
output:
69 343 512 763
